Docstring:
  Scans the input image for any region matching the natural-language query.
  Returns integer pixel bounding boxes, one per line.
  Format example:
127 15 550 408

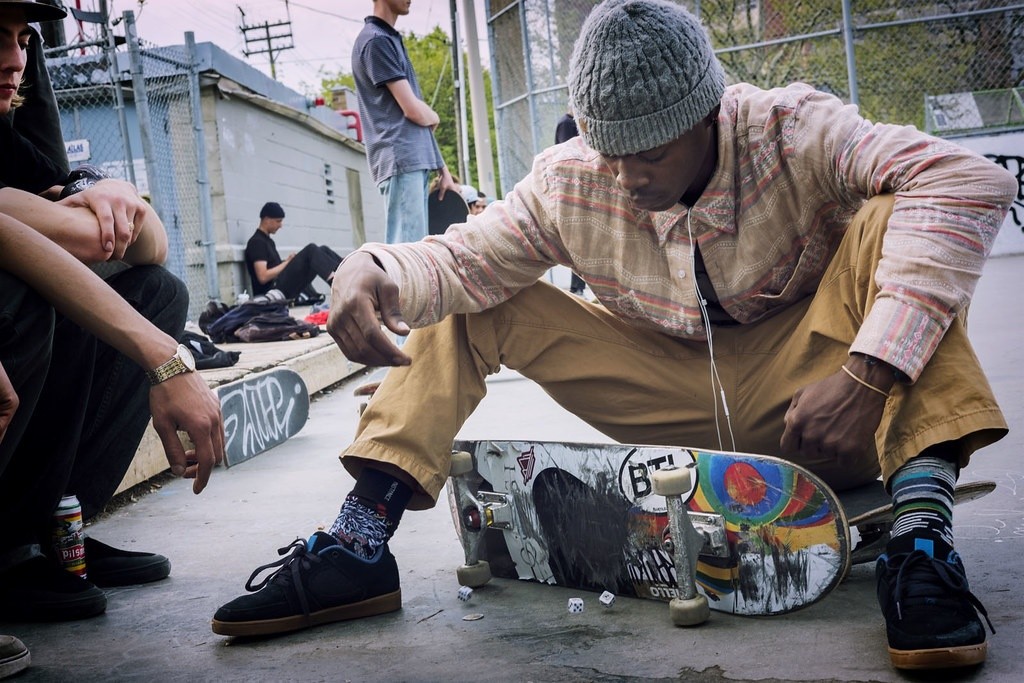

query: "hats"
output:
0 0 67 23
568 0 724 156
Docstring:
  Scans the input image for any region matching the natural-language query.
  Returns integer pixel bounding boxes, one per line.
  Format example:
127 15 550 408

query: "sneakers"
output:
875 530 996 670
0 555 107 622
80 537 172 586
212 531 401 637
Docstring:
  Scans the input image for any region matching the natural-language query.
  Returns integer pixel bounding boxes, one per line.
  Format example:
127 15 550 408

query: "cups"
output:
238 294 250 303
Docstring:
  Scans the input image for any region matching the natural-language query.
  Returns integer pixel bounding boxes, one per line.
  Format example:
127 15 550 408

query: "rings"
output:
127 222 135 230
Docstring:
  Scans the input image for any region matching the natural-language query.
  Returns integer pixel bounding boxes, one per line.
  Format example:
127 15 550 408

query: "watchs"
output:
146 343 197 385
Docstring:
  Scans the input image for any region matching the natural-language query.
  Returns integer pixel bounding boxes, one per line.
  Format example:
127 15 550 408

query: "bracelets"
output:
840 364 888 398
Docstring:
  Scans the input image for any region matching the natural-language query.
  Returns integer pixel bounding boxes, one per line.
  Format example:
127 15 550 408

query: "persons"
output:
459 181 482 217
348 0 458 249
1 211 229 683
244 202 343 301
475 191 487 214
429 170 461 197
0 0 189 629
555 98 597 302
209 0 1021 680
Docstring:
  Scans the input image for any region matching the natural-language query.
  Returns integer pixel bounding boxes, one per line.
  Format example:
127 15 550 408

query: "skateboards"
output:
445 437 851 628
210 365 310 468
427 187 471 236
835 479 997 566
